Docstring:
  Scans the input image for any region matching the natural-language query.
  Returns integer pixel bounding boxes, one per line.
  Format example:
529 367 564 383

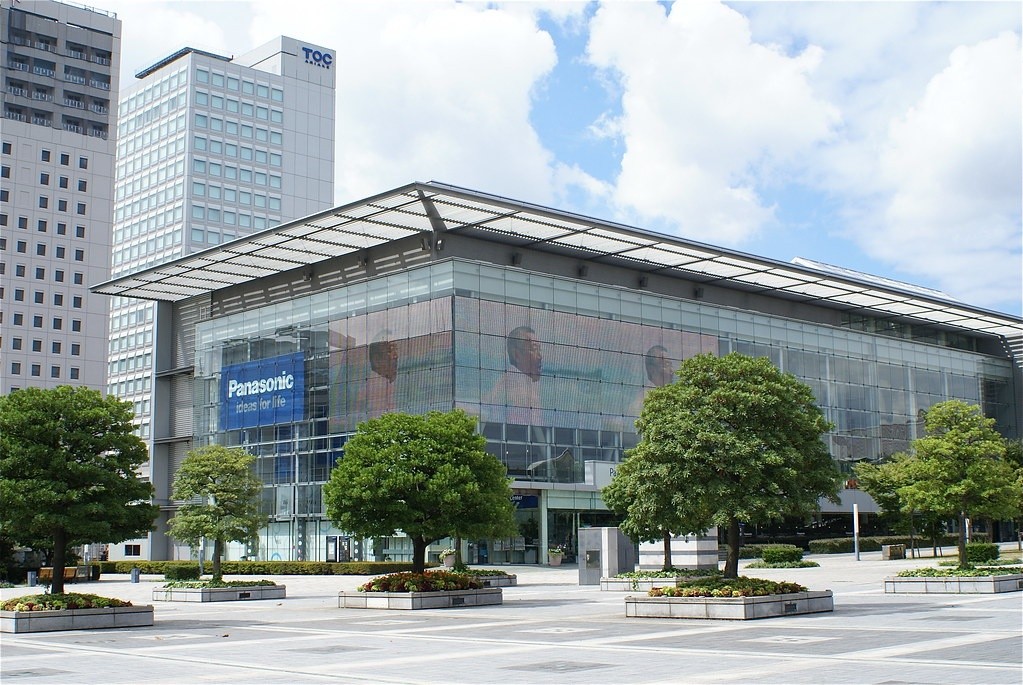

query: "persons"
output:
488 326 544 426
357 328 399 418
630 346 675 427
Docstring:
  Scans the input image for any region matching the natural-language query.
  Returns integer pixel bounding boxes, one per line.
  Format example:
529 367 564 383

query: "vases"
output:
549 555 562 566
443 555 455 567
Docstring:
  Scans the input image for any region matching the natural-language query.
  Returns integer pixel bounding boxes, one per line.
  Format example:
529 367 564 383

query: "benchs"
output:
35 567 77 586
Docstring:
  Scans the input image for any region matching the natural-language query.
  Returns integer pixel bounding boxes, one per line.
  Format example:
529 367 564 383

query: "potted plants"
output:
239 556 247 561
384 557 392 562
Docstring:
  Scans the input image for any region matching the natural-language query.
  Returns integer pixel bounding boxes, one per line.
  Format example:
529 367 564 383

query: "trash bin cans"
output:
130 563 139 583
27 571 36 587
92 565 101 580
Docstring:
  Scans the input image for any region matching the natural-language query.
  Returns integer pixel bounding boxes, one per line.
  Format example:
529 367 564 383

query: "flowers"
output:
546 549 564 560
439 549 456 562
